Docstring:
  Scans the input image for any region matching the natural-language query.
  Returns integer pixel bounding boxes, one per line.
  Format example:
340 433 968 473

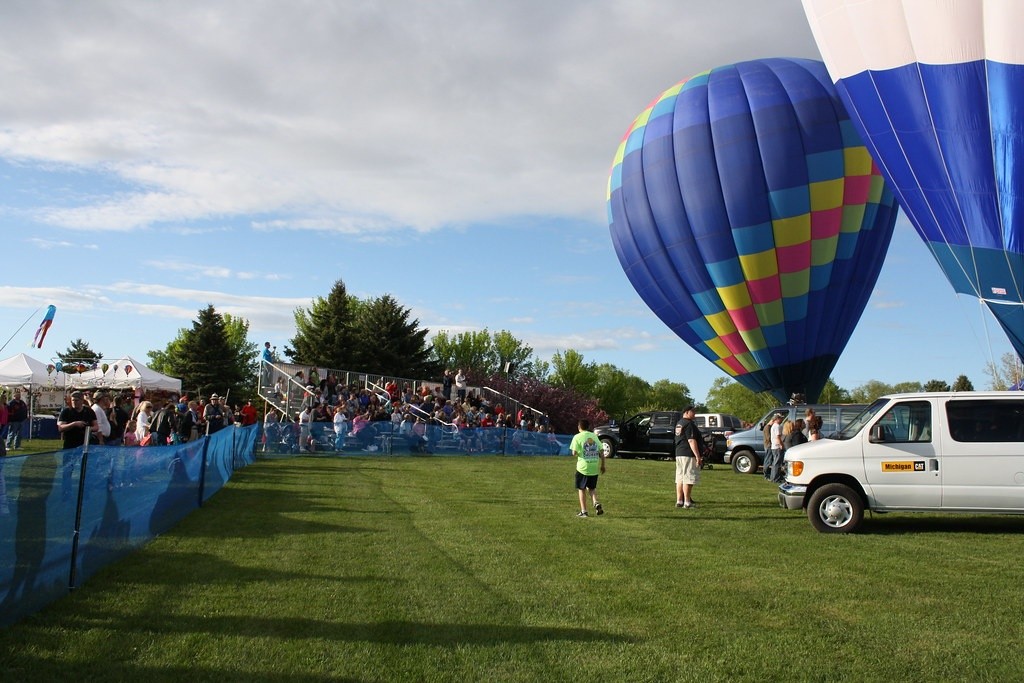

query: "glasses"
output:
812 411 816 414
268 344 270 346
146 406 152 408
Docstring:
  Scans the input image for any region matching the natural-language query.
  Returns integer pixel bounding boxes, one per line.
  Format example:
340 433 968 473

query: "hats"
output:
210 393 218 400
683 406 699 412
772 415 784 419
248 399 252 402
93 392 103 398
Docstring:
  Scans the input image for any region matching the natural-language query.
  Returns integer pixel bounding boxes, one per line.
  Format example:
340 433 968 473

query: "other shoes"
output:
262 445 559 455
774 480 781 484
93 481 137 490
765 477 771 481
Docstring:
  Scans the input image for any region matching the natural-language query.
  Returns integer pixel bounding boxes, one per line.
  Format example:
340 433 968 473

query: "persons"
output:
58 386 258 502
6 389 28 451
675 406 704 510
569 419 606 517
763 408 825 484
263 342 275 386
275 365 561 455
263 406 278 453
0 393 8 448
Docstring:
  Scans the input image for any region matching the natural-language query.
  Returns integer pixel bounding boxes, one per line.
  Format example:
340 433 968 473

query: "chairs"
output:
910 420 931 440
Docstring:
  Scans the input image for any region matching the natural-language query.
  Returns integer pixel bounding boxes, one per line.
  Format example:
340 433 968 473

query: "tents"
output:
0 352 72 439
74 355 182 400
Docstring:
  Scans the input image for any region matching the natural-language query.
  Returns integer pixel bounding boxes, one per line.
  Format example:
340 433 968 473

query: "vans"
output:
777 390 1024 534
723 401 910 474
639 413 742 429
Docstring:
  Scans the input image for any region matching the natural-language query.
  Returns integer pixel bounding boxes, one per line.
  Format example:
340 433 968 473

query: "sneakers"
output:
15 448 24 450
63 491 72 503
675 502 683 507
595 502 604 516
682 501 696 508
5 447 12 451
83 492 89 501
576 512 589 518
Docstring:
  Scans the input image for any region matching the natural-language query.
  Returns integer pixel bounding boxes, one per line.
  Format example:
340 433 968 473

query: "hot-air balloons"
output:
604 56 901 406
54 362 63 375
113 364 119 374
124 365 133 377
803 0 1024 371
101 363 109 377
46 364 55 377
76 363 85 377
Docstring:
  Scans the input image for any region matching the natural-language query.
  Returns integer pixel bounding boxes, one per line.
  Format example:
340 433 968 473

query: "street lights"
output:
503 361 514 455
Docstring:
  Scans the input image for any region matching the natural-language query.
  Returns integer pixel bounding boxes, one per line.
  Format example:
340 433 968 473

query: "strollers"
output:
272 423 300 454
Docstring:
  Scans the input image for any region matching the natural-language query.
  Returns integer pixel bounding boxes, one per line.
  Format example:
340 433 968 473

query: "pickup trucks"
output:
593 410 734 459
